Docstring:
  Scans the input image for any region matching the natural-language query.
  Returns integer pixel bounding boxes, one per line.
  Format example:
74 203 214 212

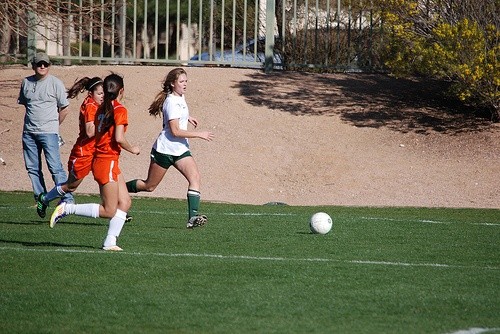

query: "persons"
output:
36 75 133 224
124 67 215 228
17 52 75 204
49 69 141 251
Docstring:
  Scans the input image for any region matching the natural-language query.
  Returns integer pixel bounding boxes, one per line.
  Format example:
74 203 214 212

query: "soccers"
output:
308 211 333 235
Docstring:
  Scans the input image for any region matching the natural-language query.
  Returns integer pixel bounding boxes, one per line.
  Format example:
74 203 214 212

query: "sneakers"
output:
103 245 123 251
37 192 50 218
186 215 208 228
125 215 132 223
50 201 67 228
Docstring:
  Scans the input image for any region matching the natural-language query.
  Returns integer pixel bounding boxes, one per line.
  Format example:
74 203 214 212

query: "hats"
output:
31 51 49 63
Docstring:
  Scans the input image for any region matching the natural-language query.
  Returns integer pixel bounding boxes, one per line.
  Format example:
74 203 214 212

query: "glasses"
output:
33 62 49 67
